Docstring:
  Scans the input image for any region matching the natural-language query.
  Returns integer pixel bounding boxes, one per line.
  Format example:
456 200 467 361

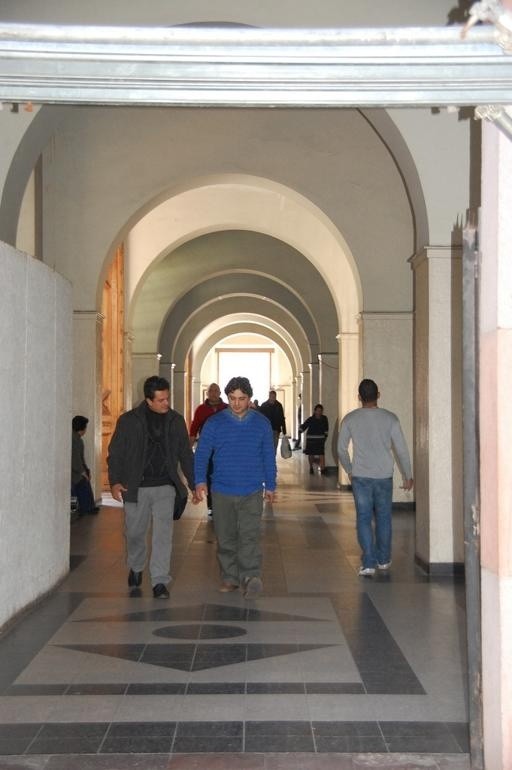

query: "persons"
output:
192 376 277 598
190 381 229 517
69 414 100 516
259 391 286 458
292 394 301 449
252 399 260 409
336 378 412 577
106 376 200 598
298 404 330 476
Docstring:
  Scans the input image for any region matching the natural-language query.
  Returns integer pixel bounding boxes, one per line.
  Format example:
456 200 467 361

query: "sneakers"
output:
357 565 375 577
242 575 264 600
128 569 142 597
152 584 170 599
378 559 392 570
218 581 238 593
